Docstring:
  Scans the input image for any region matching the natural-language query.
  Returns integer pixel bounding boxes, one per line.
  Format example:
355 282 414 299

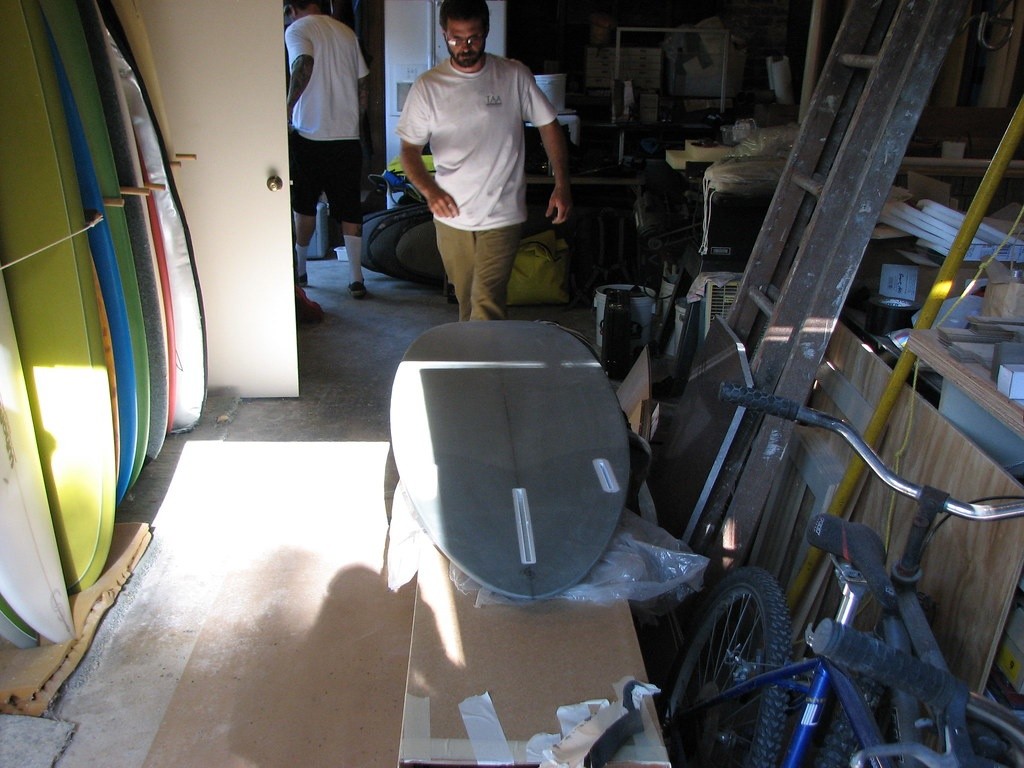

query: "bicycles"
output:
657 377 1024 768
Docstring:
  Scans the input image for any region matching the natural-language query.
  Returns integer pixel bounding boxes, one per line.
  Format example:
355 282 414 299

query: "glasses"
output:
445 32 483 46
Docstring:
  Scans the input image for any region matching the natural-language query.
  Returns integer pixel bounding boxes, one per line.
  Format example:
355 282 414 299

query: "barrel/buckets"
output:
532 72 568 111
660 276 680 323
593 283 655 349
672 297 688 353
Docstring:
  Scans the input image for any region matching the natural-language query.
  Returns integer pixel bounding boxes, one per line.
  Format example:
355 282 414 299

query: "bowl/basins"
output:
720 126 758 145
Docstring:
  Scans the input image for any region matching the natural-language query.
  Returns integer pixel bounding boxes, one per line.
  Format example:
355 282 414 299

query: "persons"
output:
284 0 370 297
393 0 571 323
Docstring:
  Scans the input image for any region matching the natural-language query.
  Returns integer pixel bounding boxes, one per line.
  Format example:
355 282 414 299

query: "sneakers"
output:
297 271 307 286
347 278 367 296
